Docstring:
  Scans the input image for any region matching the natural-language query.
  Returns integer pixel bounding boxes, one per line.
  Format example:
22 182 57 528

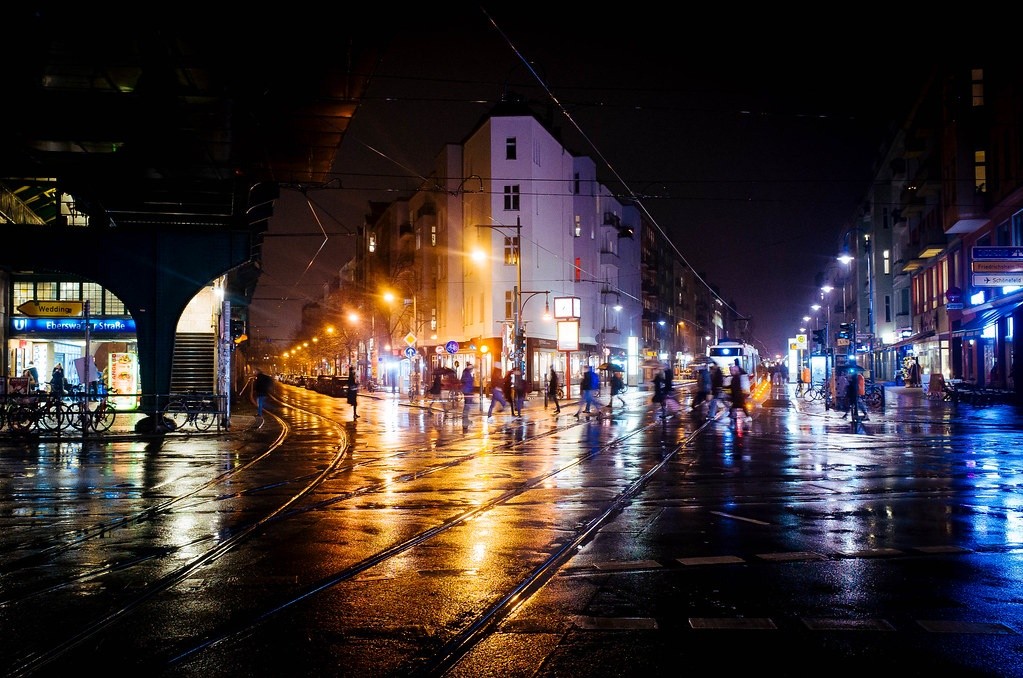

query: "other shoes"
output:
841 414 847 419
606 405 612 408
622 402 627 407
706 415 714 419
659 415 666 419
743 417 753 422
554 409 560 413
862 414 869 419
574 414 579 417
583 411 590 413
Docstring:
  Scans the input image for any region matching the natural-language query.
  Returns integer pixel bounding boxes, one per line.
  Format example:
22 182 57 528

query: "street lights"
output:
835 233 876 408
471 225 522 416
839 322 856 364
349 315 376 386
799 292 829 411
650 311 667 379
604 290 623 388
384 294 421 399
821 266 846 354
514 290 554 407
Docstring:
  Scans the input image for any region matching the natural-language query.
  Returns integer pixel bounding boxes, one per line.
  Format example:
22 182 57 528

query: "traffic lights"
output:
520 360 526 375
812 330 823 344
471 337 488 359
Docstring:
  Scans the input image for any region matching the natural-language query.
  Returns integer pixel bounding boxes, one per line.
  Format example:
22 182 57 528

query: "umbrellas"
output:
687 356 717 364
597 363 625 378
431 367 455 375
841 364 866 371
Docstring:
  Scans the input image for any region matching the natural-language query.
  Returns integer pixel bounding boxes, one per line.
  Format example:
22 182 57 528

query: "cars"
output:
278 373 350 397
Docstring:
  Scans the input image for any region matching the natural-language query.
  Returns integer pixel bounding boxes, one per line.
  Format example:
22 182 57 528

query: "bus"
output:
705 338 762 395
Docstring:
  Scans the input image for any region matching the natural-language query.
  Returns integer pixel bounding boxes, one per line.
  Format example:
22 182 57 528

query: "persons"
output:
991 362 1002 393
461 363 475 425
651 365 675 411
52 363 64 392
547 364 560 413
21 361 38 390
761 362 788 387
606 371 625 407
686 358 753 430
908 362 918 388
487 367 521 423
428 375 450 413
573 365 603 417
347 367 360 419
89 363 99 402
253 368 271 417
848 369 870 420
803 366 811 390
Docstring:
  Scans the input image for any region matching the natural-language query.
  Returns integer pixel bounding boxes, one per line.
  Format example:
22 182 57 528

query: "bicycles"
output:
422 385 433 399
796 377 881 409
162 391 216 432
448 387 463 402
539 381 564 400
0 385 116 431
408 385 418 403
619 384 628 393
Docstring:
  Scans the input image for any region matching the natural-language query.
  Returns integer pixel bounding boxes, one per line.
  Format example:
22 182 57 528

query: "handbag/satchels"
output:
620 380 624 389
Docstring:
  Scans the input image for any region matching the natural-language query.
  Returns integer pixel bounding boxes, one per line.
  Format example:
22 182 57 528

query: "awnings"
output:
891 330 935 348
951 301 1023 337
